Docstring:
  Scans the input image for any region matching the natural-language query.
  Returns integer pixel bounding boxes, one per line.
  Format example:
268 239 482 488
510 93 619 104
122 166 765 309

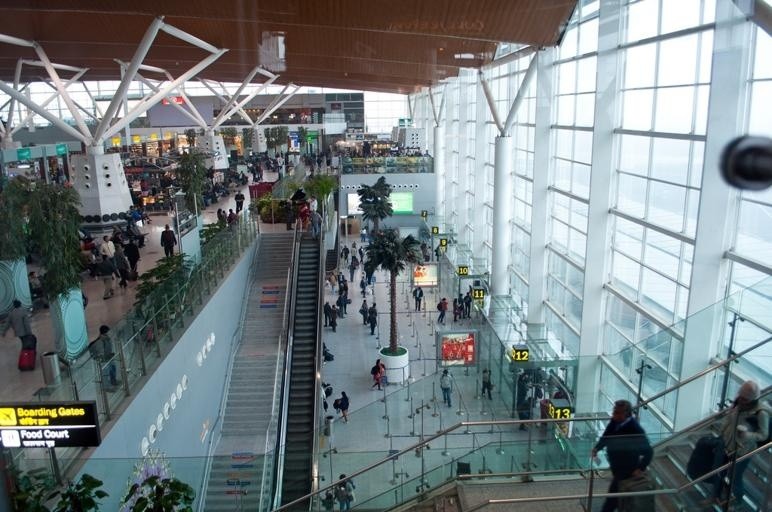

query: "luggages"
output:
618 473 656 512
686 436 729 484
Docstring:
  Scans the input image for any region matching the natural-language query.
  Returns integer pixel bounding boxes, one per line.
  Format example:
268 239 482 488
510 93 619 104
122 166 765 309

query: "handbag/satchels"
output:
755 409 772 448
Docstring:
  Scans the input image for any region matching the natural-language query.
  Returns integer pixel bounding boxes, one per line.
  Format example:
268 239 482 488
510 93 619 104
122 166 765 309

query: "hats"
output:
739 381 761 400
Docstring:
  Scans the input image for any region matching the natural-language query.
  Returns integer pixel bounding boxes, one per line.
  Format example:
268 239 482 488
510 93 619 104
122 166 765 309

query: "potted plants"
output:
359 222 426 384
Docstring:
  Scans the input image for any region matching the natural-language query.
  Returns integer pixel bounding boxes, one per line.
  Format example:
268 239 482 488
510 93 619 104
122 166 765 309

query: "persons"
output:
1 187 179 386
360 277 367 298
440 369 453 407
324 271 348 332
697 379 771 511
438 292 473 324
341 241 365 282
338 392 349 421
371 359 385 389
360 227 367 242
324 474 354 510
413 287 423 311
178 186 323 240
517 368 564 431
482 368 492 400
421 241 440 262
54 143 431 187
362 300 377 335
588 399 655 512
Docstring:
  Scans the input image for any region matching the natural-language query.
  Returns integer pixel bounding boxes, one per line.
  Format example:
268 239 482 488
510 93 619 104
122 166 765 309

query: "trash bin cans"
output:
324 415 335 436
41 350 62 395
